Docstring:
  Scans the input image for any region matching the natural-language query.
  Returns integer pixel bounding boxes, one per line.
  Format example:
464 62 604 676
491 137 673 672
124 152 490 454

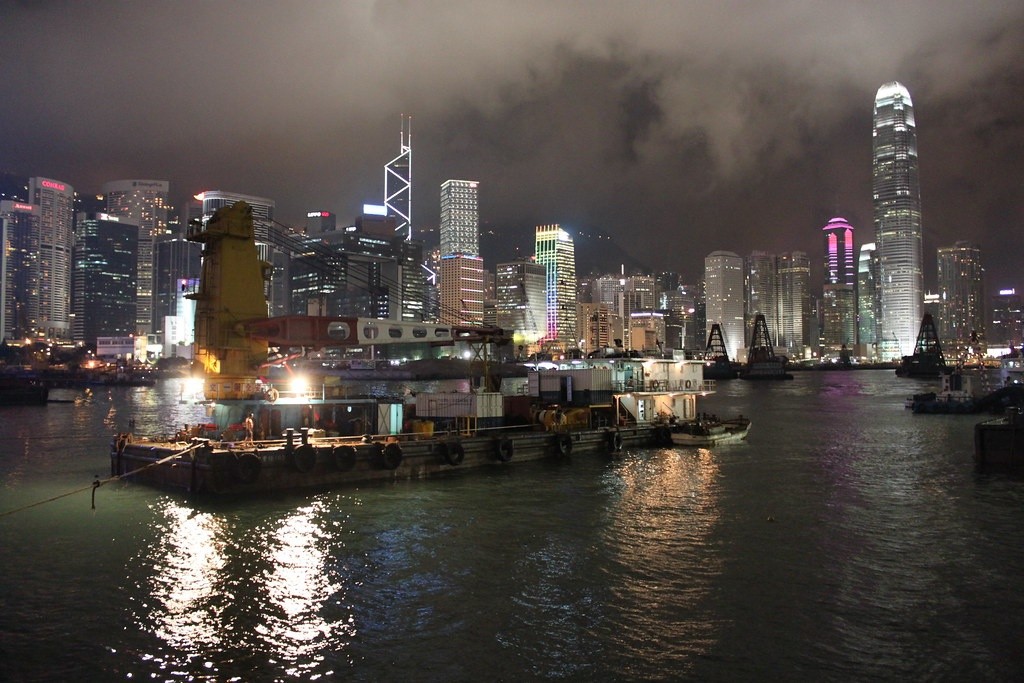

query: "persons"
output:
244 413 255 447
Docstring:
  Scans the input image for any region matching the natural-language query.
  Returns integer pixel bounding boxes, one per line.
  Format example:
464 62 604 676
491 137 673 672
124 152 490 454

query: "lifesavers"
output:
686 380 691 389
610 432 623 451
234 452 264 483
446 443 465 465
292 445 319 472
266 388 279 402
557 433 573 456
331 445 358 472
653 380 659 389
494 438 514 462
380 442 404 470
658 427 672 444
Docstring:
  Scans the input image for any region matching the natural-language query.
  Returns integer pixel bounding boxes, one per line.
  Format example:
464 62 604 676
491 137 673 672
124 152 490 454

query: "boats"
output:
739 315 794 380
895 312 948 378
671 418 752 446
702 324 739 379
110 199 715 500
